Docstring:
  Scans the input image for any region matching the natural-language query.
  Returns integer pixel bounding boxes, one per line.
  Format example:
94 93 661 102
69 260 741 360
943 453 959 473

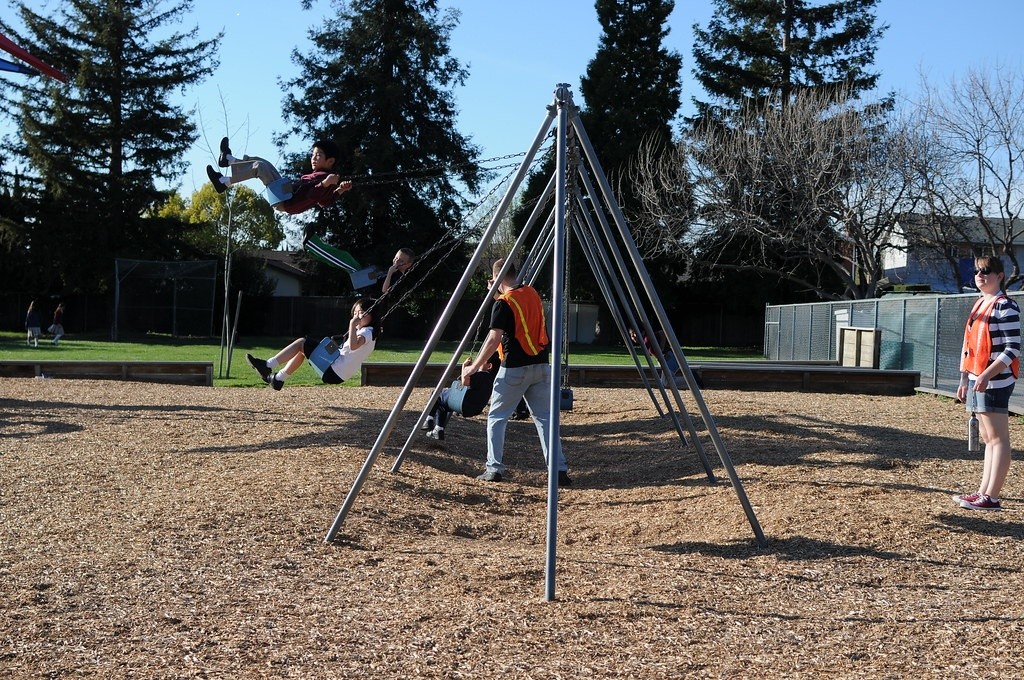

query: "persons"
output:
471 278 530 421
26 301 42 348
953 255 1021 510
47 303 65 345
463 256 573 486
206 136 353 215
629 323 679 387
302 221 415 299
420 350 501 441
246 300 381 391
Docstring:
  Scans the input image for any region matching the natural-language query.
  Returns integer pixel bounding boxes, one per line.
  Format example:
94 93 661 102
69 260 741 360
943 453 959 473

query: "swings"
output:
559 201 573 411
448 209 555 416
308 130 556 380
574 202 680 374
349 185 555 290
266 145 554 208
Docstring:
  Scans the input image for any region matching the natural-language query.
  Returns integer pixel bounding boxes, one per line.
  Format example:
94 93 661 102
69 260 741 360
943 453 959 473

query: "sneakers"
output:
421 419 435 430
245 354 273 379
953 491 985 503
426 427 445 440
558 470 571 485
960 494 1002 511
207 165 229 194
476 468 502 481
219 136 232 167
264 372 284 390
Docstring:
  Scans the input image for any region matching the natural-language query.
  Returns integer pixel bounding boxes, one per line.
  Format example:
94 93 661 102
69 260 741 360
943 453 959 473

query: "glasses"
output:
972 267 994 275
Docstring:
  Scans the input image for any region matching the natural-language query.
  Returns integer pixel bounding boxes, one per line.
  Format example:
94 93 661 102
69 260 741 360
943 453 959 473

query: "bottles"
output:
967 413 980 452
